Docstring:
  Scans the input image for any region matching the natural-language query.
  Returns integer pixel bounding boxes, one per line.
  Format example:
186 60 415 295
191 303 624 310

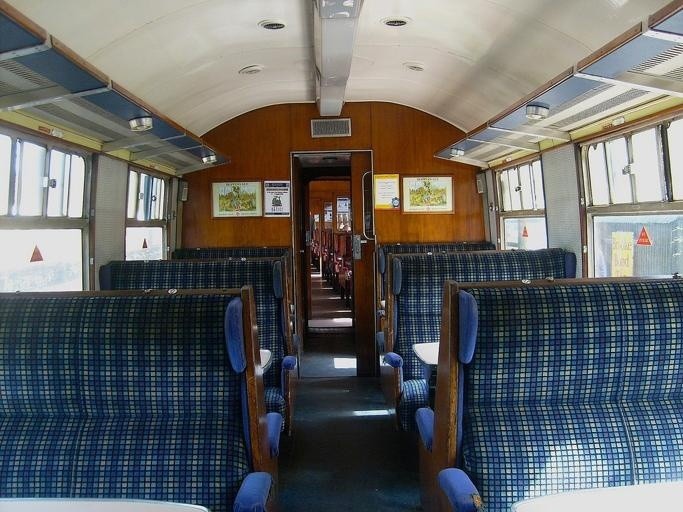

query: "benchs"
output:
0 286 283 510
171 246 301 354
376 241 496 353
311 228 352 308
433 278 682 512
383 248 576 442
100 257 298 461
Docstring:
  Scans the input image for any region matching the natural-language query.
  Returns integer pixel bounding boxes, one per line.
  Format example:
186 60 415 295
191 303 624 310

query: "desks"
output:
2 498 211 511
511 478 683 511
411 341 439 365
259 349 274 376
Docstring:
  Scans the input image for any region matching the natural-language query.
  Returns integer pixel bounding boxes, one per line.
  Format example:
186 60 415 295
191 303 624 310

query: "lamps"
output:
129 116 153 133
526 105 551 120
450 148 466 158
203 154 216 165
312 0 363 116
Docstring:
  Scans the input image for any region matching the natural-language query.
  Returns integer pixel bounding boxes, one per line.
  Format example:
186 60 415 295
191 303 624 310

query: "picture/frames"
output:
400 173 456 215
209 179 263 219
